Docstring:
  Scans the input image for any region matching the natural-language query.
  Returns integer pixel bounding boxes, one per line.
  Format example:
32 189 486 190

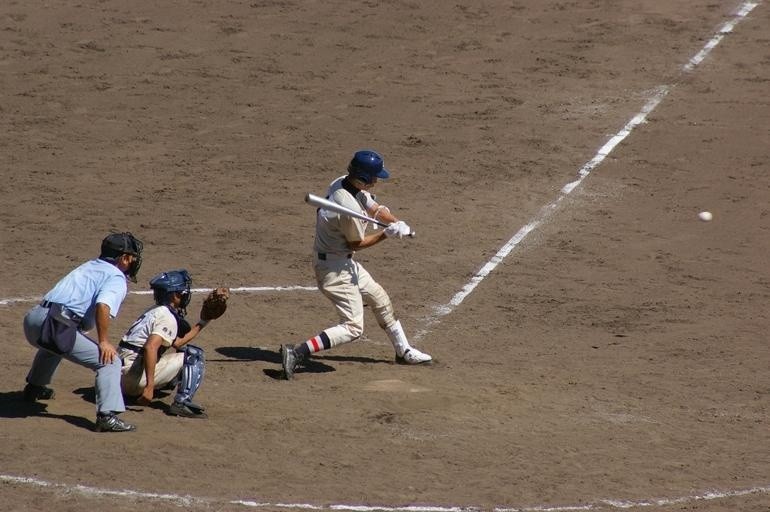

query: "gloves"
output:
383 222 399 238
396 221 412 238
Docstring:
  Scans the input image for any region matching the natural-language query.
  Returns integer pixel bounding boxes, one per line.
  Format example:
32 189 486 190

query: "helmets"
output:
346 150 390 181
153 270 191 294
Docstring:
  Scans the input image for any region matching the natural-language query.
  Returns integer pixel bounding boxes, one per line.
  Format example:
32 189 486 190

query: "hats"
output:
97 233 136 260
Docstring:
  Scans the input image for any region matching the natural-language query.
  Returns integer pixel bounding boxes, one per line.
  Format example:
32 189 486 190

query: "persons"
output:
21 231 146 434
118 269 231 419
281 148 433 381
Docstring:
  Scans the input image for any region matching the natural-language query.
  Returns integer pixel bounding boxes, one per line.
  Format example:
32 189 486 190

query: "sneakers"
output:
280 343 301 380
23 384 56 399
169 401 210 419
95 412 137 432
395 347 433 364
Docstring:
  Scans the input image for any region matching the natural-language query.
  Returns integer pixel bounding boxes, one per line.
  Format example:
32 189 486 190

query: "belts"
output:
317 253 353 261
118 339 146 355
40 299 52 309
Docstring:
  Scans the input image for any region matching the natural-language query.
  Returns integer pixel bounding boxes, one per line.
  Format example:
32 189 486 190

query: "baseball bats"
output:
303 191 416 237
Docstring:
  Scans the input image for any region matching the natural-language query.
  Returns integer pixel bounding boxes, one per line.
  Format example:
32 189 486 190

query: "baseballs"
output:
697 209 713 225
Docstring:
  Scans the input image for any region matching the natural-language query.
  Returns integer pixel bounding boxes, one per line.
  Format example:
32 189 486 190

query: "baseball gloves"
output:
199 284 230 317
